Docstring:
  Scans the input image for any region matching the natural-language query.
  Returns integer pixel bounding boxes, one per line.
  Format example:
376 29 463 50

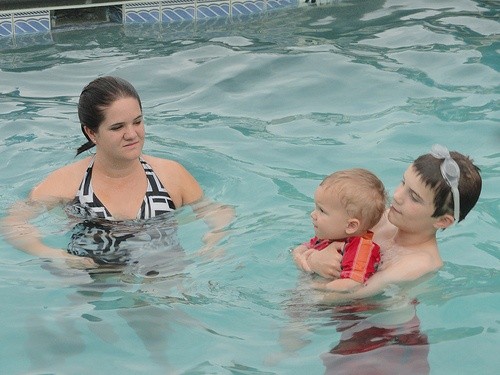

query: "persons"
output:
263 144 483 374
3 76 236 374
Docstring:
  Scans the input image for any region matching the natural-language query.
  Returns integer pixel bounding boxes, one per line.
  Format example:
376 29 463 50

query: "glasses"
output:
431 144 460 221
